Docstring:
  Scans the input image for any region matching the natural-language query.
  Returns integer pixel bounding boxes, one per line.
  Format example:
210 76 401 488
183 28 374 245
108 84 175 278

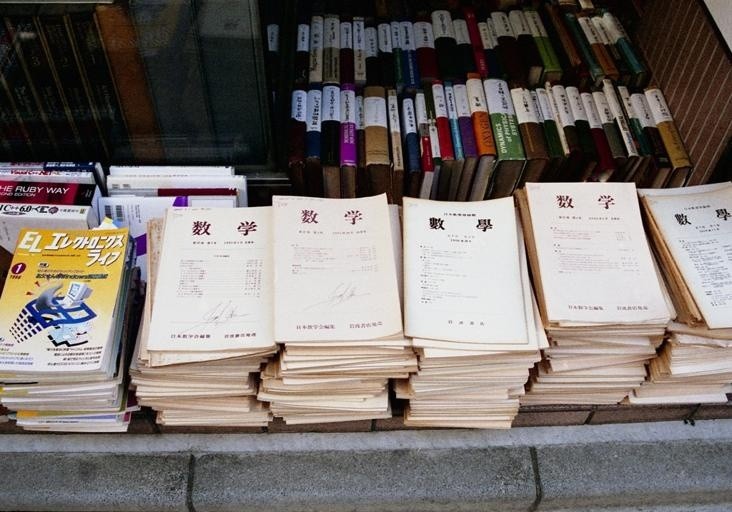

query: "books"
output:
0 0 273 177
98 166 248 228
0 228 147 432
257 182 732 429
263 0 693 206
0 163 107 254
129 206 281 427
